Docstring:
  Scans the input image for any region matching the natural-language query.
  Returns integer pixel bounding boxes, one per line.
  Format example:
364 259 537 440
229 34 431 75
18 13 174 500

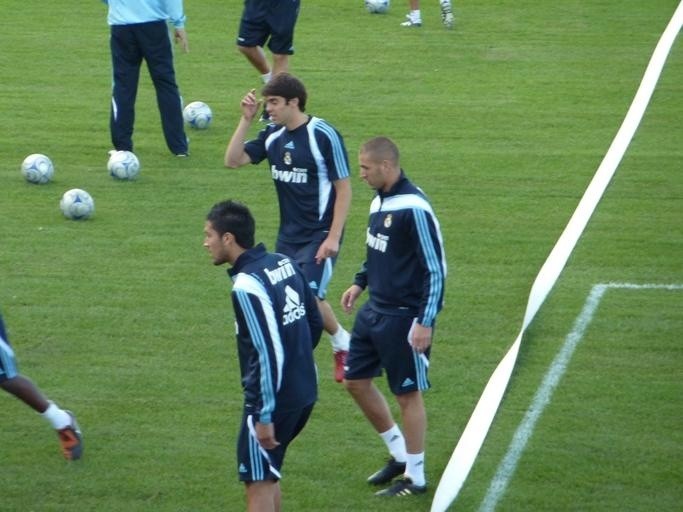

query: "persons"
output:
339 135 446 499
103 1 194 156
235 0 297 119
200 201 322 511
0 316 82 460
399 1 455 29
224 71 353 383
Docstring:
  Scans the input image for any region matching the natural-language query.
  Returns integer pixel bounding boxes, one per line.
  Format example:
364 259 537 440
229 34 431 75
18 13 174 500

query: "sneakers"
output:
400 16 419 27
57 409 83 460
368 461 406 486
441 0 454 27
258 105 271 120
376 478 427 499
335 334 352 382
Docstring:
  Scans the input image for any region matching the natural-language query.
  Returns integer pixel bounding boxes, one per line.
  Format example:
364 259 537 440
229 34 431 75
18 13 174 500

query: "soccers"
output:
365 0 390 14
106 151 140 180
21 155 53 184
183 101 212 129
59 188 94 220
